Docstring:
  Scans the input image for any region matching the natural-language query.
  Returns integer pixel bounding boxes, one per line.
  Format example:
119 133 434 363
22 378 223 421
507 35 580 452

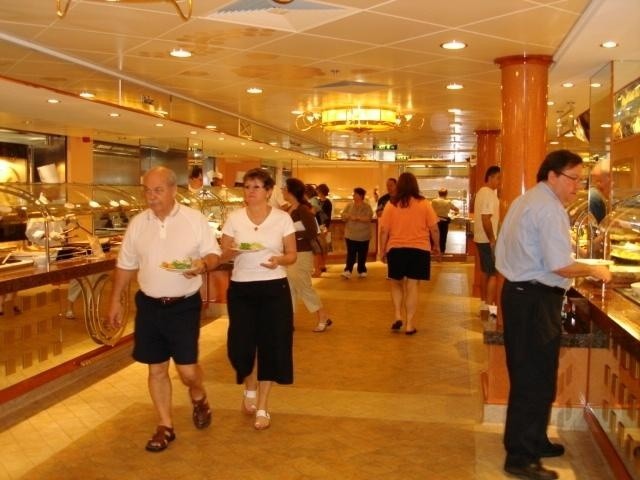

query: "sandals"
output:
254 410 270 430
192 396 211 429
146 425 175 451
241 389 257 414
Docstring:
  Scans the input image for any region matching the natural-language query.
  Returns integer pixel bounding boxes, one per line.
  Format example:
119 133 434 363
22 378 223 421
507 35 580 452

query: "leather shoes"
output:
540 442 564 458
504 462 557 480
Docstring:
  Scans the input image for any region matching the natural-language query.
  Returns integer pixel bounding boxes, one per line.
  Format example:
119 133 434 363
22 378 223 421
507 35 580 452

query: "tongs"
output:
57 225 79 241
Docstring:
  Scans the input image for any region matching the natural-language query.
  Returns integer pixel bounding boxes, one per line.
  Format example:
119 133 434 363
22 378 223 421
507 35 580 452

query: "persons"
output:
210 171 236 221
220 166 298 431
378 172 442 335
472 165 501 319
280 177 332 334
375 178 397 218
494 149 613 479
340 187 373 279
269 184 333 277
430 187 459 256
108 164 223 451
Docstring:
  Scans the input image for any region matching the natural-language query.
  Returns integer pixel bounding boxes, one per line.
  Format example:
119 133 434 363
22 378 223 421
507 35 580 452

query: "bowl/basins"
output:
31 253 47 268
630 282 640 298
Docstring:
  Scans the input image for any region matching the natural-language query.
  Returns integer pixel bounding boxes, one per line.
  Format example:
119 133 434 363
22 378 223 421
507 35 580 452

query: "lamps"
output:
294 107 426 135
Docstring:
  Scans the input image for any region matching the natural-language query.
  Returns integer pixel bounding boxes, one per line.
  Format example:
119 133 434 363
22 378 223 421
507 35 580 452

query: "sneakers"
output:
341 272 352 279
360 273 368 278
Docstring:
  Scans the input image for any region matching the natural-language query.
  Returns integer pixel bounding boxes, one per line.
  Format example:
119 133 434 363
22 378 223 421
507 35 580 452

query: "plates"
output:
227 245 266 254
164 266 198 274
574 257 614 267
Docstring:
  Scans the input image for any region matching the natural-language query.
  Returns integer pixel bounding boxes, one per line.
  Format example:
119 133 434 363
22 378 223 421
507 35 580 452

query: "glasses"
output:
560 172 584 183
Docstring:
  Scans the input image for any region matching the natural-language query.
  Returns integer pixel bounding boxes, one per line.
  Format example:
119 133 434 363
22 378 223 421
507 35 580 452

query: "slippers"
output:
405 328 416 334
312 319 332 332
392 320 402 329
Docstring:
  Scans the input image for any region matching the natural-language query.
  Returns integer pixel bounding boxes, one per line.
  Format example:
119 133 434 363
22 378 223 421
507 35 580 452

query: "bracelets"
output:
201 257 208 273
